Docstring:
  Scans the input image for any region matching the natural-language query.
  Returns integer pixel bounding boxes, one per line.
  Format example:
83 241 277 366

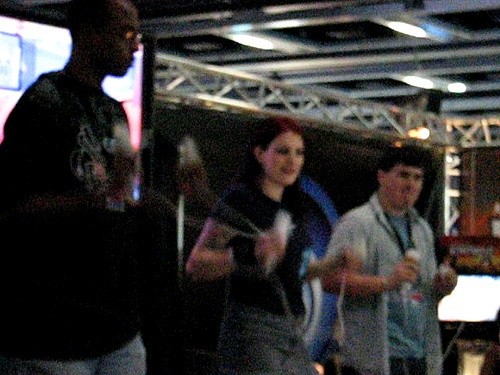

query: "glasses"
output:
94 24 143 43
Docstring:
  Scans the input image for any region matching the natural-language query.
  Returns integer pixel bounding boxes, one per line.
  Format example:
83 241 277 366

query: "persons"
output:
0 0 146 374
321 145 456 374
185 115 332 375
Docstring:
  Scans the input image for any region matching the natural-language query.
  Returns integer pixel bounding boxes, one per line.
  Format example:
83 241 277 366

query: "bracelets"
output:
227 234 258 270
